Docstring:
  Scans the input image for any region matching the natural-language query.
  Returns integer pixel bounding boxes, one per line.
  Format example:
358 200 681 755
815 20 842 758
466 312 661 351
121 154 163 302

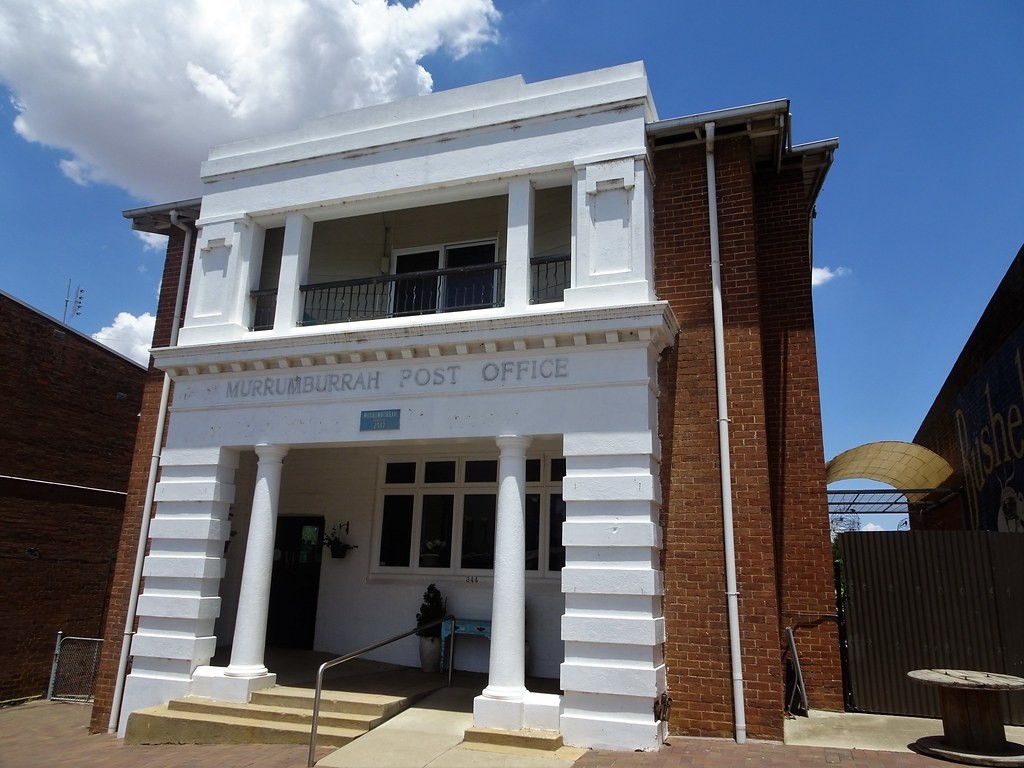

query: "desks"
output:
907 669 1024 768
439 618 492 674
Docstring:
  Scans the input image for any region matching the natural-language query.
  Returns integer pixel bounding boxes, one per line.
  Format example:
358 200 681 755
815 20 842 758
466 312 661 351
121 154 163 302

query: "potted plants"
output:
415 583 447 673
321 530 350 559
